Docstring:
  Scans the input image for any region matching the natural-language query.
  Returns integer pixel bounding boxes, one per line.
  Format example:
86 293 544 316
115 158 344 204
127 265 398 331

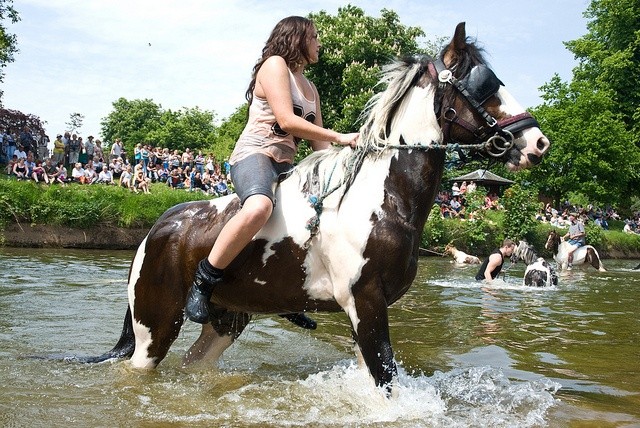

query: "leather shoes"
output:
279 313 317 330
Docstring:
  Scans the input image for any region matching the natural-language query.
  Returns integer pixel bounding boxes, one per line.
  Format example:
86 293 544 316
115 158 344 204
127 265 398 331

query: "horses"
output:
17 19 551 401
509 229 607 289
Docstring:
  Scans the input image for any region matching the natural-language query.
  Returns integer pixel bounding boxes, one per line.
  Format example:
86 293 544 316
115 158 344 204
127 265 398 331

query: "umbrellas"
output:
448 169 517 187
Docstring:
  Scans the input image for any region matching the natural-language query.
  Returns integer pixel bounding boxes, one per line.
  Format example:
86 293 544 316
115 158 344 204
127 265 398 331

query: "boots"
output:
184 259 223 324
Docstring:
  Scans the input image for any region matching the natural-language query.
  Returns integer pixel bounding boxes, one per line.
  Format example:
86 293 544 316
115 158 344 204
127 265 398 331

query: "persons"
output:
562 214 585 270
475 239 515 282
434 180 504 221
0 126 233 196
536 199 640 234
186 17 361 330
